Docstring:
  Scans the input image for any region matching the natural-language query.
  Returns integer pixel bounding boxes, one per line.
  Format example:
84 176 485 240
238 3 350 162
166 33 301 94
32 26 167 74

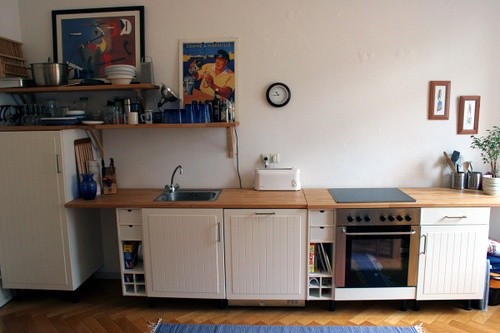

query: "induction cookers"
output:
328 187 416 202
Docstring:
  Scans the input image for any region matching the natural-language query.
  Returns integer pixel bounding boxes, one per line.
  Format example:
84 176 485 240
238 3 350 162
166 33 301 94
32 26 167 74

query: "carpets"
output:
150 319 425 333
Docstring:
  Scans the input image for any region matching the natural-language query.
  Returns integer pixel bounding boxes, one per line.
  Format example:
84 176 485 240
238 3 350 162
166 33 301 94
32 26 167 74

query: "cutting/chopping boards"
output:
74 137 96 181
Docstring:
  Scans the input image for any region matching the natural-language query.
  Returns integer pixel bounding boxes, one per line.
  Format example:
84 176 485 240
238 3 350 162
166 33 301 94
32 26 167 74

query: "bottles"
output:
213 87 221 122
221 98 228 121
80 172 97 200
124 97 131 113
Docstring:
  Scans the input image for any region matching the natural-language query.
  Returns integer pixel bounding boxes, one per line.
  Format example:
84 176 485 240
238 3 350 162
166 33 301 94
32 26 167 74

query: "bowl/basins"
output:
104 63 136 85
65 109 85 123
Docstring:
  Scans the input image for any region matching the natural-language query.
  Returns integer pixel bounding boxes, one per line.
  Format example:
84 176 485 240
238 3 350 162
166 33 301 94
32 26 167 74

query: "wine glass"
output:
28 103 35 125
21 103 32 125
33 103 40 124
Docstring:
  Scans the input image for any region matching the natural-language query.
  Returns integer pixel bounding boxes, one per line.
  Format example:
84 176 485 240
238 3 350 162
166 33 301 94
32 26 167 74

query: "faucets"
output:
171 165 183 192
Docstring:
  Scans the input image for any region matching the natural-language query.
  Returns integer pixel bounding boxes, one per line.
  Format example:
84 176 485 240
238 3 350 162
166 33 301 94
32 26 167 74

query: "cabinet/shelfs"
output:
1 128 104 292
142 207 226 300
222 206 308 301
116 207 145 297
416 207 490 301
307 208 336 300
0 81 241 159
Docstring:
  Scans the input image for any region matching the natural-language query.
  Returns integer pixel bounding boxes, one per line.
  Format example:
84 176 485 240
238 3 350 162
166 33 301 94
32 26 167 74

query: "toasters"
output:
254 166 302 192
335 208 422 287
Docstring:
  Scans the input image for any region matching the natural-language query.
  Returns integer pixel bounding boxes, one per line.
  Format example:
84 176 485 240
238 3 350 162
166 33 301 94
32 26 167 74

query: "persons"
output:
189 49 235 99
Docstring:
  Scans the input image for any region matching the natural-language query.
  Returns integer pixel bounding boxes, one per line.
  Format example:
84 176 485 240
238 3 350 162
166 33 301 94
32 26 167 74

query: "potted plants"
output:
471 126 500 195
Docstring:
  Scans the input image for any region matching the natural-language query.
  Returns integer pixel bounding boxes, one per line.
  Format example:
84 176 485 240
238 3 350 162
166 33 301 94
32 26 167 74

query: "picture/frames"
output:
458 95 481 134
51 6 145 84
175 34 243 110
428 81 451 120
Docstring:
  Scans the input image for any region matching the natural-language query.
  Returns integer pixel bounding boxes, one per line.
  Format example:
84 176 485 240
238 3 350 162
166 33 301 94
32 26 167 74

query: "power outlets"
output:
270 152 280 163
261 153 271 163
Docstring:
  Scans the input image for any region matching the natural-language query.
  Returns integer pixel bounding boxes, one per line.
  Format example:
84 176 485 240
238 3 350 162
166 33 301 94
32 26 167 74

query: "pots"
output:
29 62 64 85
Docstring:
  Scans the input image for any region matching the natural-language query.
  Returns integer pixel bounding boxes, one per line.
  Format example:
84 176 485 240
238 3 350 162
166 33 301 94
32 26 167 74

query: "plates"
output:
82 119 104 124
41 116 79 124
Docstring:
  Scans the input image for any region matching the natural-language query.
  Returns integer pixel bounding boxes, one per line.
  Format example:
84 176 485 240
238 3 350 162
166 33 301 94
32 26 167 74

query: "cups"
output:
451 171 465 189
127 111 138 124
140 109 154 123
467 171 482 189
183 99 213 122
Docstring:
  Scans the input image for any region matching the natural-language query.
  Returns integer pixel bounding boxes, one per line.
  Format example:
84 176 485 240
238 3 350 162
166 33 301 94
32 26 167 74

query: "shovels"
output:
452 151 460 165
462 160 470 173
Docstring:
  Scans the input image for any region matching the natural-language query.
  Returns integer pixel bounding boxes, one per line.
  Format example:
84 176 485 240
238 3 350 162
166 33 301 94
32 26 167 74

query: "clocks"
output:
266 83 291 107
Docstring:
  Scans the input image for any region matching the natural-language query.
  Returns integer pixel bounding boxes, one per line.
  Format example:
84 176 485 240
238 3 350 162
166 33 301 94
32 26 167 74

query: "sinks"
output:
153 190 222 202
